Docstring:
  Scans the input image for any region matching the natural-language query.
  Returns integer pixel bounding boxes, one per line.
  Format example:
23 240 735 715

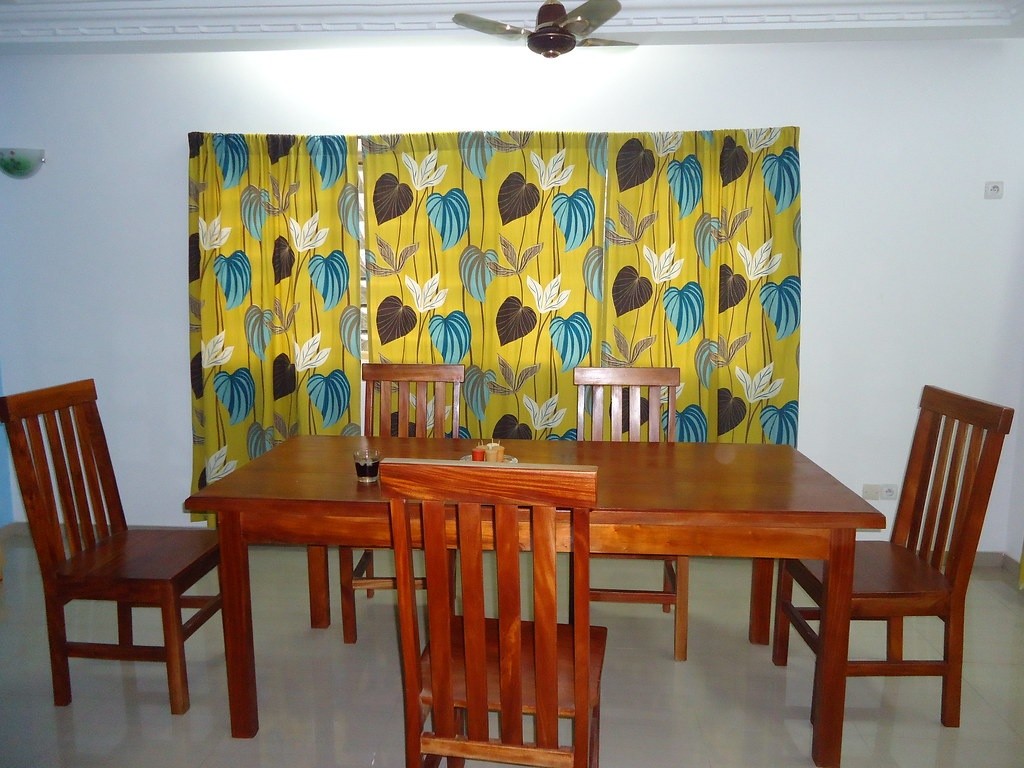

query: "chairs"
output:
0 377 230 717
378 458 610 766
567 367 690 662
339 362 466 645
771 384 1016 730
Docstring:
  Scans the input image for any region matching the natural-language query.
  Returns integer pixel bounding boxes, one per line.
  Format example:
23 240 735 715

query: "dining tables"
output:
180 434 881 767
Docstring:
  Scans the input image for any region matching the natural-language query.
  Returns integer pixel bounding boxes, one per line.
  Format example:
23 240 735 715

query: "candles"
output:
472 438 505 463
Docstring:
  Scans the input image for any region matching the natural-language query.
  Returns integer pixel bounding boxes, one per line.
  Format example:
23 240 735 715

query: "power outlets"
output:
862 484 898 502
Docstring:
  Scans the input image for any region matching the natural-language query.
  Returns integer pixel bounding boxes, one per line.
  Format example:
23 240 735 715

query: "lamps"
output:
0 146 45 178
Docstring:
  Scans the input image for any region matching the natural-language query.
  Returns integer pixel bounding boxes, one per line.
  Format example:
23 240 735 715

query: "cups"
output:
353 448 382 483
471 442 505 462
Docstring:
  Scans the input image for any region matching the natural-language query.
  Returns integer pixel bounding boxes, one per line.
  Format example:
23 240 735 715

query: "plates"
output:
459 454 518 464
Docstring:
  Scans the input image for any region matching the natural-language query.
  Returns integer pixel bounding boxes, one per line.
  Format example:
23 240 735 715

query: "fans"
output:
452 1 641 59
